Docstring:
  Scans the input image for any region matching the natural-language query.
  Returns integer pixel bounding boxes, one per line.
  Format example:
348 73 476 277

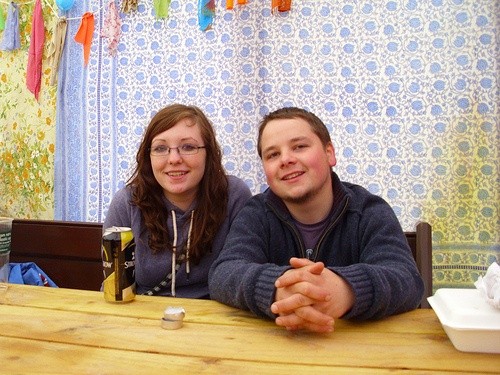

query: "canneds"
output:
102 226 137 303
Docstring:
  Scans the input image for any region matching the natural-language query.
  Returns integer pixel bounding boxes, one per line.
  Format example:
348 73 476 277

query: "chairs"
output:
11 219 104 292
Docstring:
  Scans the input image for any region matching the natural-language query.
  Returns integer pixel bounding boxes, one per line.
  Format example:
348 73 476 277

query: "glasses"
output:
146 144 206 157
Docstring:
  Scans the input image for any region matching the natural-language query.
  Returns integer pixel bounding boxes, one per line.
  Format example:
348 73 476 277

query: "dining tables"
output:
0 279 500 375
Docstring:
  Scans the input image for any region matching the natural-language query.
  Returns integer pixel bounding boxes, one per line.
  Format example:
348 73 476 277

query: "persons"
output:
100 103 251 302
207 107 425 336
